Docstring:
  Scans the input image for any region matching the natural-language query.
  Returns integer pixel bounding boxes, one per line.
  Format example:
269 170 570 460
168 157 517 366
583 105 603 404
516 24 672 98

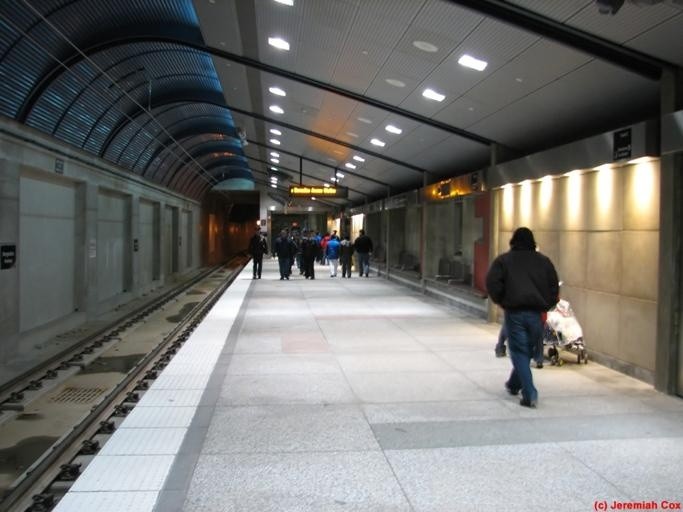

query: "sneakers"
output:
280 275 290 280
331 275 369 278
253 275 262 279
300 272 315 279
505 381 536 407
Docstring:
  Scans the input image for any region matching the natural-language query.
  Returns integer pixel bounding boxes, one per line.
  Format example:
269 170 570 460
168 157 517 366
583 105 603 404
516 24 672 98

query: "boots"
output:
496 346 507 357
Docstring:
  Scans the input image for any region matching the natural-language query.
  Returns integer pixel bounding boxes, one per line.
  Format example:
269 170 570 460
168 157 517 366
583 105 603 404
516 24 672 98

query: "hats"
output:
510 227 537 244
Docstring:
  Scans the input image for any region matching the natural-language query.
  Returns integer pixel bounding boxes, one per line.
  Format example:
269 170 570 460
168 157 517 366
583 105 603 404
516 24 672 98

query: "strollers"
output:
543 299 588 366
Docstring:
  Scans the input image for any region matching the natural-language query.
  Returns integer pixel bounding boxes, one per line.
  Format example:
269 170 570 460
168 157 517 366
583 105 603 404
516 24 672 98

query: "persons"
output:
288 201 293 208
249 226 268 279
486 227 559 406
355 230 373 277
272 230 353 280
